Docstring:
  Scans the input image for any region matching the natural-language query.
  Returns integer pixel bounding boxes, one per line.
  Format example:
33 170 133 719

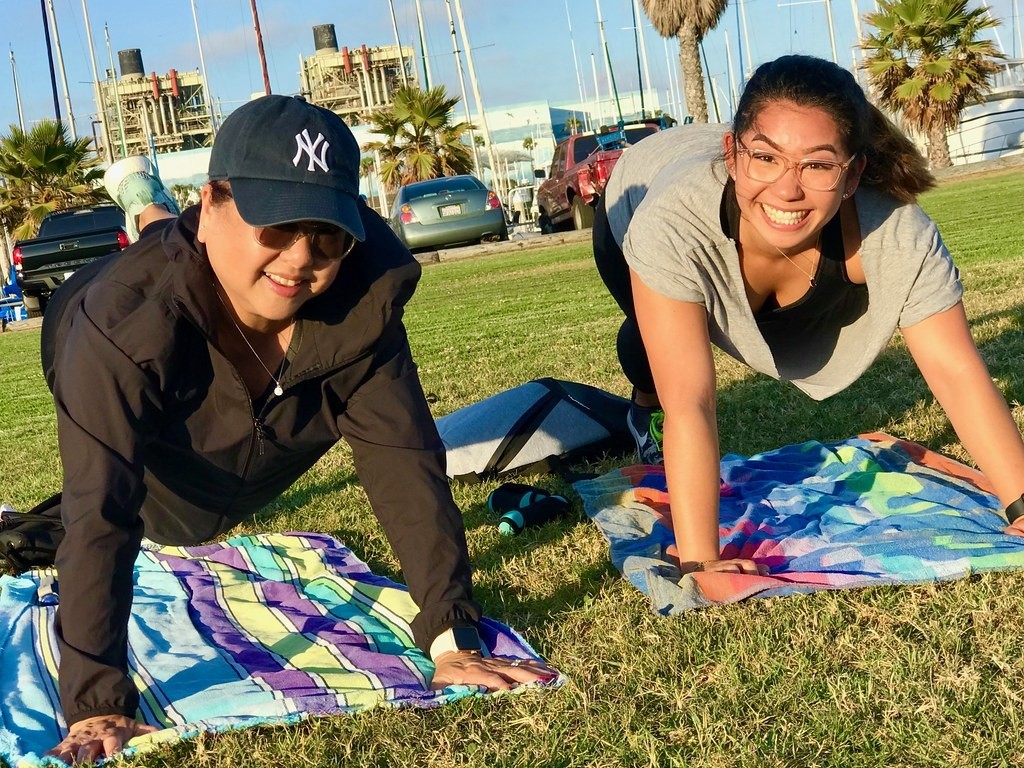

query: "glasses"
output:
210 182 355 262
736 131 858 192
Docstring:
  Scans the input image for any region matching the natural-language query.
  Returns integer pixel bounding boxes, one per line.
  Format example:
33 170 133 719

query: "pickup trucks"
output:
13 201 129 316
532 122 660 234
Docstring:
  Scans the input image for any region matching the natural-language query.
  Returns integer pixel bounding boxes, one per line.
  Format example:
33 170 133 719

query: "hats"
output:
209 95 365 242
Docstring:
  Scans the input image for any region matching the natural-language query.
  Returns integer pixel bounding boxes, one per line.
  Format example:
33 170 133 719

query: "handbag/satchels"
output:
435 377 631 484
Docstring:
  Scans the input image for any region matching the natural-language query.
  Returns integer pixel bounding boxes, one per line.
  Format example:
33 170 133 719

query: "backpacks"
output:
0 492 66 575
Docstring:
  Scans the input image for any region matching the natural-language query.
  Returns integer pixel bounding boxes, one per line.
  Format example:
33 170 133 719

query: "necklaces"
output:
773 238 819 289
212 282 297 396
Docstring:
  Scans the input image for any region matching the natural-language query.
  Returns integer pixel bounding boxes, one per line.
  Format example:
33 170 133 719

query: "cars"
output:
0 297 27 323
387 174 509 253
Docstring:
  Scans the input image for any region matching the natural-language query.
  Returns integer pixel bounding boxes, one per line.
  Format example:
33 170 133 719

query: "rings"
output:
510 658 522 667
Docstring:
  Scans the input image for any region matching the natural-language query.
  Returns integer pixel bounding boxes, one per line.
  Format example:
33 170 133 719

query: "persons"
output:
40 95 563 766
591 54 1024 577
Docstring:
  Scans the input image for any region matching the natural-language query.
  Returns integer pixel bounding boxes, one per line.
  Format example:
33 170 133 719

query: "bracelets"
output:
1005 492 1024 525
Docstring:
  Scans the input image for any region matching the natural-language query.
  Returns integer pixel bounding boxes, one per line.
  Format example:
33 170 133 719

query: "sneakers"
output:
627 402 664 466
103 156 180 243
